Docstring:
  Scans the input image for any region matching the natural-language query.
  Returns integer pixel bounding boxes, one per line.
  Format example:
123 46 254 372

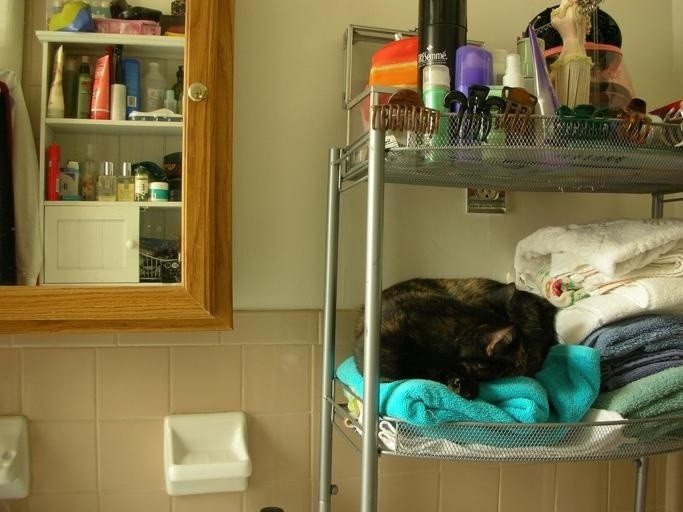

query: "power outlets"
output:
465 186 508 214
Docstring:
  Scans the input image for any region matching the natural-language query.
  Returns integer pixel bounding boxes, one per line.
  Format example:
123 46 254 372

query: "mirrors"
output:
0 0 236 338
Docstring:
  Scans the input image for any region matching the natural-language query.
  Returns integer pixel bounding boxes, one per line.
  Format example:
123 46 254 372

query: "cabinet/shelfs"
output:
29 27 185 286
319 102 682 512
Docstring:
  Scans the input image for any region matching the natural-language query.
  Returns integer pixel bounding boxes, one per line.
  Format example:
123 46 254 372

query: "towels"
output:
334 345 638 458
514 217 682 446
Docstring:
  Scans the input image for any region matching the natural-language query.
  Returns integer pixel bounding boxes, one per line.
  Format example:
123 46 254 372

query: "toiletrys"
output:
44 142 185 201
47 42 185 123
417 1 563 167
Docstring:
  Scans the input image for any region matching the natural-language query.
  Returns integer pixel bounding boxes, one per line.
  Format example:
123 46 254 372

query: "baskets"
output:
138 252 180 282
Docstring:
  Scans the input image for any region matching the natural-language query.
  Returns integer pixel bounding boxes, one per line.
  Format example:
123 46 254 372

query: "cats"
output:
352 276 558 401
